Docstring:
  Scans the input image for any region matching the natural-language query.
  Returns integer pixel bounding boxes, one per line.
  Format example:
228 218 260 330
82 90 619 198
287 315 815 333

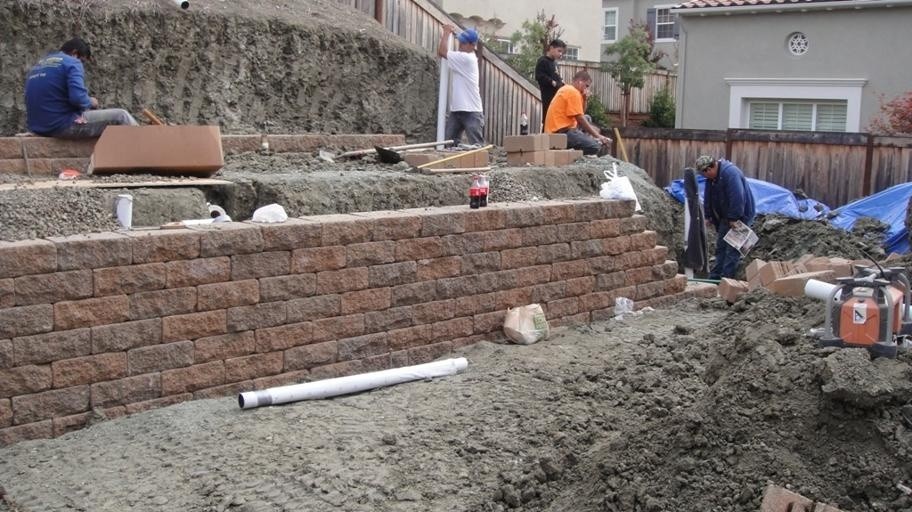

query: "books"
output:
723 220 759 258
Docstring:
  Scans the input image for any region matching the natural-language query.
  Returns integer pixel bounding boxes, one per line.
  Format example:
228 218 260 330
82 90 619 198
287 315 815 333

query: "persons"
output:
536 39 566 134
437 23 487 147
543 71 612 158
697 156 757 283
25 38 140 139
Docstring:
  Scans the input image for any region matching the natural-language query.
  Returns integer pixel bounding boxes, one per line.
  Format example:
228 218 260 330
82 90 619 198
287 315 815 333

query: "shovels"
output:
375 145 403 163
319 139 454 164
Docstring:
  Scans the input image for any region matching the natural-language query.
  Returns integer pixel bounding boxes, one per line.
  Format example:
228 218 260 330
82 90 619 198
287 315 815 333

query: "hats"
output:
455 28 478 44
696 155 714 171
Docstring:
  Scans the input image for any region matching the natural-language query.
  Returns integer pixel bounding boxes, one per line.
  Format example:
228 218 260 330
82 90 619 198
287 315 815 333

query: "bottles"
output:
520 112 528 136
469 176 488 209
